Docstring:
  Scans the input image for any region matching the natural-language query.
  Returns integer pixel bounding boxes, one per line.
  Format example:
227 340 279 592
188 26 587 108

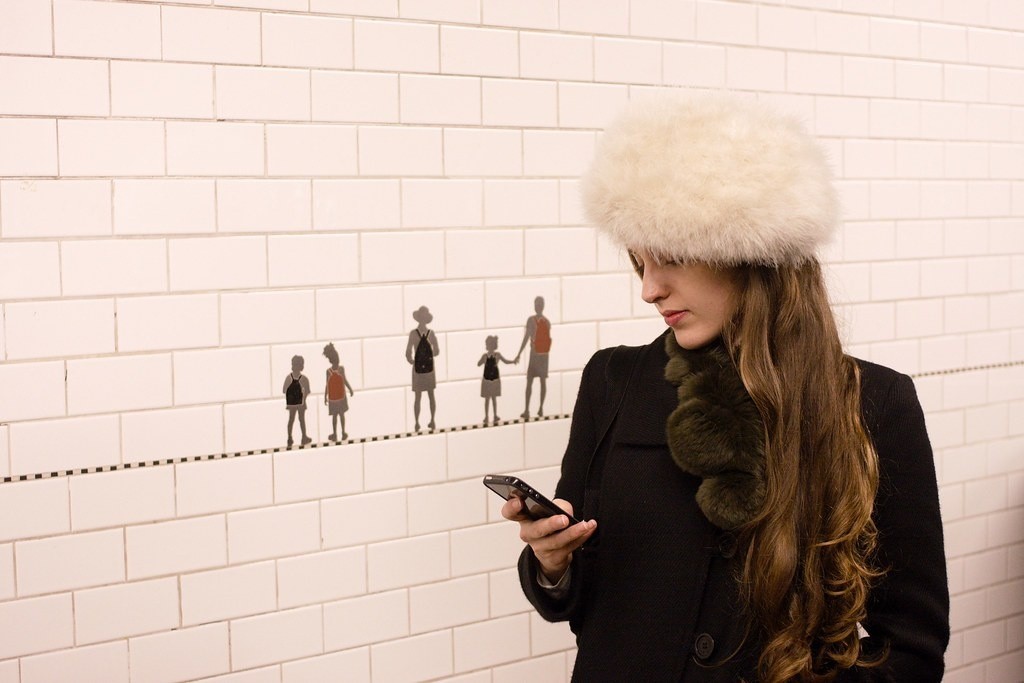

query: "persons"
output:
501 114 951 683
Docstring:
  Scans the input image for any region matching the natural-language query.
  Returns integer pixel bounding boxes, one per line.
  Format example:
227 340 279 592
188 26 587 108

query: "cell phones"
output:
483 474 581 535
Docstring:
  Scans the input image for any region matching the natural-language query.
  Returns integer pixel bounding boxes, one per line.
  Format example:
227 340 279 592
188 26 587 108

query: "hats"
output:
581 93 837 266
